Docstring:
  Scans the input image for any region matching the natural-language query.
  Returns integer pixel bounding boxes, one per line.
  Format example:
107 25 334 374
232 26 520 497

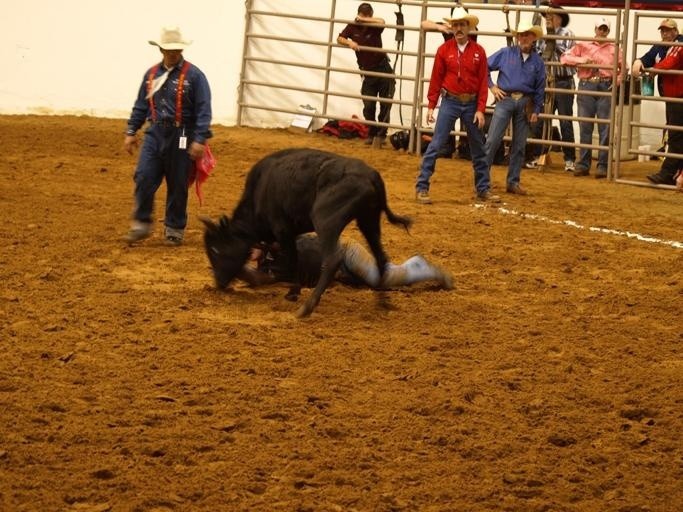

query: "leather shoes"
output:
646 172 673 185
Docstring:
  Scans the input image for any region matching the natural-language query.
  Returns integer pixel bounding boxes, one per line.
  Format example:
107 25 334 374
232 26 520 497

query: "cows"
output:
196 147 415 320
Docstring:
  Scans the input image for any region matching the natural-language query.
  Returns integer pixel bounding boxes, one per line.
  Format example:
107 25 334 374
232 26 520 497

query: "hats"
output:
443 6 478 32
360 3 372 15
148 27 191 51
541 5 568 28
657 19 677 30
595 17 610 31
509 20 543 42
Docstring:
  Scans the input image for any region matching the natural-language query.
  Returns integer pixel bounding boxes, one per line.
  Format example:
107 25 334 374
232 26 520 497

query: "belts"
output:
554 75 573 80
446 93 477 103
581 77 610 84
507 91 524 101
152 120 194 128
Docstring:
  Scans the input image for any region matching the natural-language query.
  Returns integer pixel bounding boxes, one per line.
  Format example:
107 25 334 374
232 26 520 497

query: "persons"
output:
631 18 683 185
236 233 455 292
560 16 638 178
525 2 577 173
413 6 501 204
117 25 212 247
485 22 547 195
421 6 473 161
336 2 397 146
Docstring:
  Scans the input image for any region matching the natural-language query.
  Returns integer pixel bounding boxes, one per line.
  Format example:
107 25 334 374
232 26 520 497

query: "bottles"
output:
640 72 654 97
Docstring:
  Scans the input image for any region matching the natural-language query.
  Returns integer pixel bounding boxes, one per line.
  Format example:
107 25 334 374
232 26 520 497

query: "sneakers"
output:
506 183 526 195
525 157 538 169
364 134 372 144
564 161 574 172
476 189 500 202
164 213 187 241
127 220 149 243
415 189 430 204
574 164 589 176
372 135 382 150
595 164 606 178
